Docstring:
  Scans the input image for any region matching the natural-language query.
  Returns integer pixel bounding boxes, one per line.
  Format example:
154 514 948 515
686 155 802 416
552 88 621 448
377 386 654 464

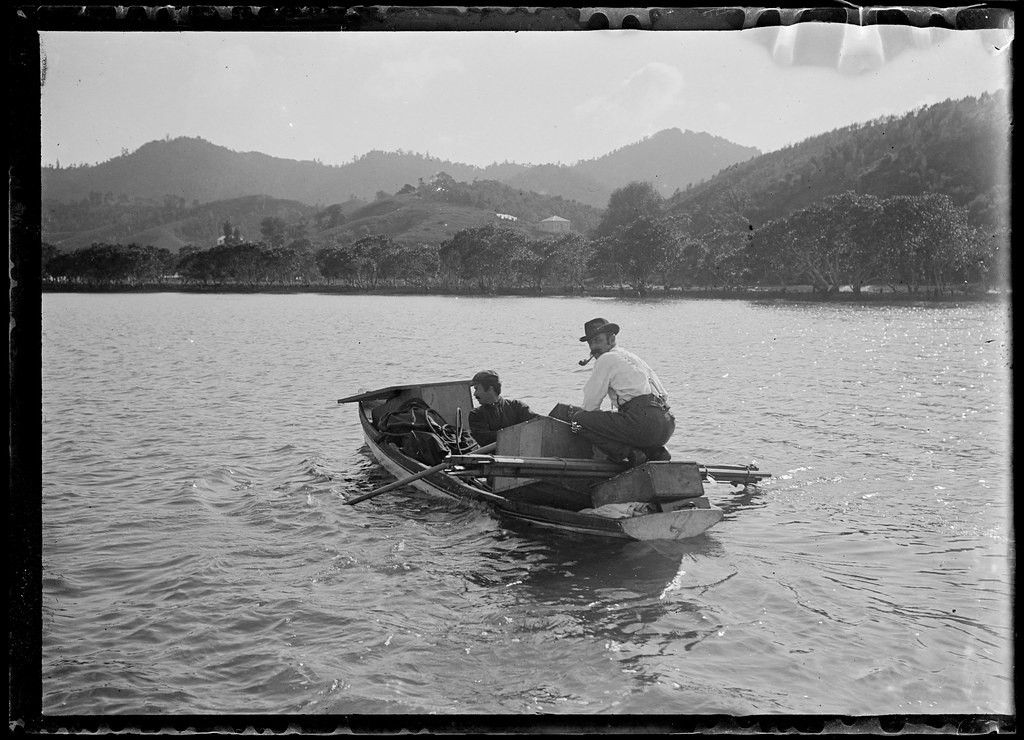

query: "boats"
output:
338 380 724 541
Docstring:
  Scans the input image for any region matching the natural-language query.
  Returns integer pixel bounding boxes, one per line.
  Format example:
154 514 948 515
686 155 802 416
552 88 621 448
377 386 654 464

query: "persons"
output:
467 370 542 455
571 317 675 468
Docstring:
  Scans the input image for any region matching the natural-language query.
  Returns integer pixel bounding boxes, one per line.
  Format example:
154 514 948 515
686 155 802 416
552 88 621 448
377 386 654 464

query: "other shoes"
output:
624 448 648 471
647 447 671 462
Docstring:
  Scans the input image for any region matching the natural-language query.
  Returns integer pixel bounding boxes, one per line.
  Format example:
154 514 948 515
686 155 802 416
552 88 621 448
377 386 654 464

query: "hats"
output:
469 370 500 386
580 318 620 343
